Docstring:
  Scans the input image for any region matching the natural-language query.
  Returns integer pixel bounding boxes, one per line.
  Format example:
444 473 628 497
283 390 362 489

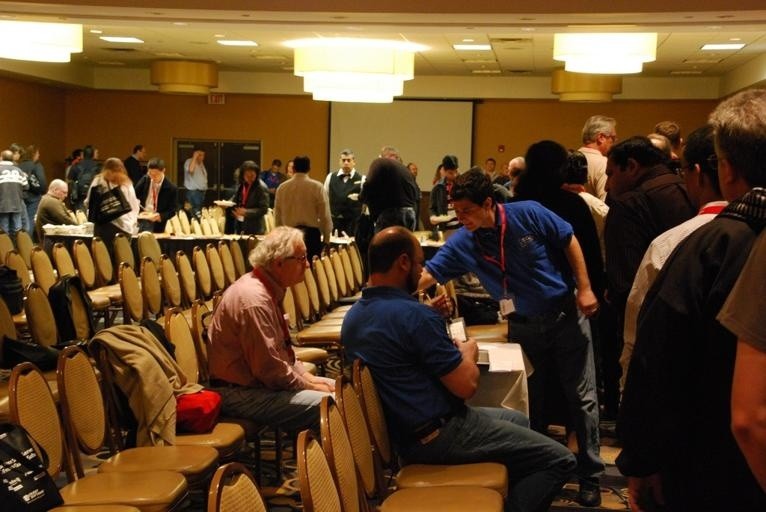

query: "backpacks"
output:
72 163 100 205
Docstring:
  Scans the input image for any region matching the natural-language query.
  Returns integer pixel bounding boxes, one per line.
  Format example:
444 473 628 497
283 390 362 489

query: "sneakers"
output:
578 479 602 506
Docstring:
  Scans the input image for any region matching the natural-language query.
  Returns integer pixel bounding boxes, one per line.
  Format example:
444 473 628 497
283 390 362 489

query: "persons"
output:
2 144 181 262
323 149 366 237
354 147 403 280
646 120 684 163
339 224 579 512
272 154 333 263
416 166 605 505
279 160 294 184
260 159 282 209
407 161 420 232
429 155 463 229
215 161 269 234
510 87 765 511
615 87 765 511
203 224 338 447
485 157 525 192
184 145 207 220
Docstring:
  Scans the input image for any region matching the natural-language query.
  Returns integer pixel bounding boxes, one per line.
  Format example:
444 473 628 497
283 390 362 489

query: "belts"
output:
209 375 242 389
412 411 453 444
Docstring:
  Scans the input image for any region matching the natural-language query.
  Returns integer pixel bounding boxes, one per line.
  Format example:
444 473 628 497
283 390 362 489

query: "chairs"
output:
0 206 510 511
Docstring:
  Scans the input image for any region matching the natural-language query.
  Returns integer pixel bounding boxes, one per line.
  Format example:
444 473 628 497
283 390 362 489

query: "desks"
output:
456 342 527 414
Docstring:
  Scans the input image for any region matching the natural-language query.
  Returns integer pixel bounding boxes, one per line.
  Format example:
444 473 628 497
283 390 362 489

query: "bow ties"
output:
343 174 350 178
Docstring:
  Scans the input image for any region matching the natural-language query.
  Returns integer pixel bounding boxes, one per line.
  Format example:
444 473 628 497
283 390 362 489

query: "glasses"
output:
286 255 306 263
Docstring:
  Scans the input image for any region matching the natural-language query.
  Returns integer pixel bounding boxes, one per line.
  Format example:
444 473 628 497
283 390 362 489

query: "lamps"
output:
0 18 749 103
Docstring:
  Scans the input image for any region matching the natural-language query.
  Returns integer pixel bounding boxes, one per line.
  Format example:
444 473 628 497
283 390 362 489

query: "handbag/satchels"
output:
88 185 101 220
0 421 66 511
28 171 42 192
100 186 131 221
175 387 222 432
